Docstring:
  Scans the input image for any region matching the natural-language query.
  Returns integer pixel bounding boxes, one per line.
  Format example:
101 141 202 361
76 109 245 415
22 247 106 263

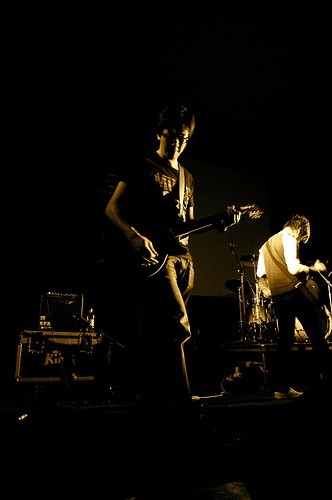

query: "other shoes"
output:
320 371 332 384
274 386 304 400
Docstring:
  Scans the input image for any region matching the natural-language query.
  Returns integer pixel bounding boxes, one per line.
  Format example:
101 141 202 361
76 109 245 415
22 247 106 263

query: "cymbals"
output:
224 279 256 295
240 254 258 267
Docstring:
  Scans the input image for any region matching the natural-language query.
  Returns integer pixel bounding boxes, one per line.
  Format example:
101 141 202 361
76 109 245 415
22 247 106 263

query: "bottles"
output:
87 307 94 328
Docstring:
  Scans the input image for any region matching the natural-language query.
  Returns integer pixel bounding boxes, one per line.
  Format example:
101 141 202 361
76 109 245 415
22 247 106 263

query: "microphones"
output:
228 240 235 255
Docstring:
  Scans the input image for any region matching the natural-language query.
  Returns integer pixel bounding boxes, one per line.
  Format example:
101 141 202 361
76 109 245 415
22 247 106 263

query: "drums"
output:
276 305 332 342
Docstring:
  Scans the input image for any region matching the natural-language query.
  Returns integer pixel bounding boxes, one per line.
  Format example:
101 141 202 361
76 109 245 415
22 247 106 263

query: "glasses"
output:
159 132 187 143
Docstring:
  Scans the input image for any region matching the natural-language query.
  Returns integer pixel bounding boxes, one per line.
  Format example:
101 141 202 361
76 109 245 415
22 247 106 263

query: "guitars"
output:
302 279 320 301
101 201 264 279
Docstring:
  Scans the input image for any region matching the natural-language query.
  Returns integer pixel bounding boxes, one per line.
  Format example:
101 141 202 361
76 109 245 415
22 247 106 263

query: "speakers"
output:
38 291 85 333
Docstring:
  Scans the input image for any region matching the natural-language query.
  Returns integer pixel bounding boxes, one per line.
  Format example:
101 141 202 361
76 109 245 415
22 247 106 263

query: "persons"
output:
256 215 332 399
106 106 242 405
